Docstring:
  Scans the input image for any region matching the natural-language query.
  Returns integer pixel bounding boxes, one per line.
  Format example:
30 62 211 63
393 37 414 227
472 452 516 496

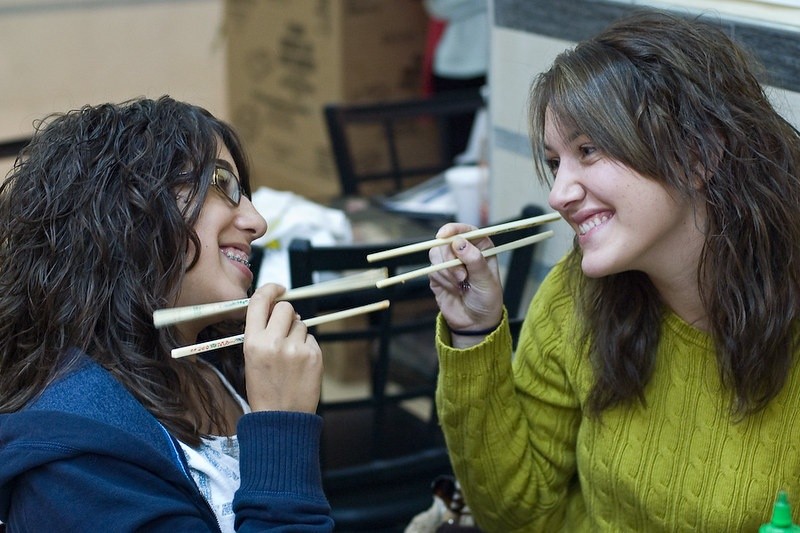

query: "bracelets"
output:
448 327 498 336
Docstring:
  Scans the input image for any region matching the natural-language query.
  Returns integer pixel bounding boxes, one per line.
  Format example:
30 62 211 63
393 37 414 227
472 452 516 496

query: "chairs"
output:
200 91 547 533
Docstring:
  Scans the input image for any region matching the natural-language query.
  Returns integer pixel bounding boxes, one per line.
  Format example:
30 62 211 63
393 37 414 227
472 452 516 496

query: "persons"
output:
429 13 800 533
0 95 343 532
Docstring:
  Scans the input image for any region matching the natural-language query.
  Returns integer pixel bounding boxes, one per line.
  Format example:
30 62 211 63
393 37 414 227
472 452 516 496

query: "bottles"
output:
758 491 800 533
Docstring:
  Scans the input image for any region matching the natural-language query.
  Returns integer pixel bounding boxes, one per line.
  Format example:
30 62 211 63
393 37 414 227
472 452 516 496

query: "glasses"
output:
177 166 247 205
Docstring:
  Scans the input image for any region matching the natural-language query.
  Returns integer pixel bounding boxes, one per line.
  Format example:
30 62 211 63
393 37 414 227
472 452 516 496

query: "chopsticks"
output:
366 210 561 288
152 265 390 360
